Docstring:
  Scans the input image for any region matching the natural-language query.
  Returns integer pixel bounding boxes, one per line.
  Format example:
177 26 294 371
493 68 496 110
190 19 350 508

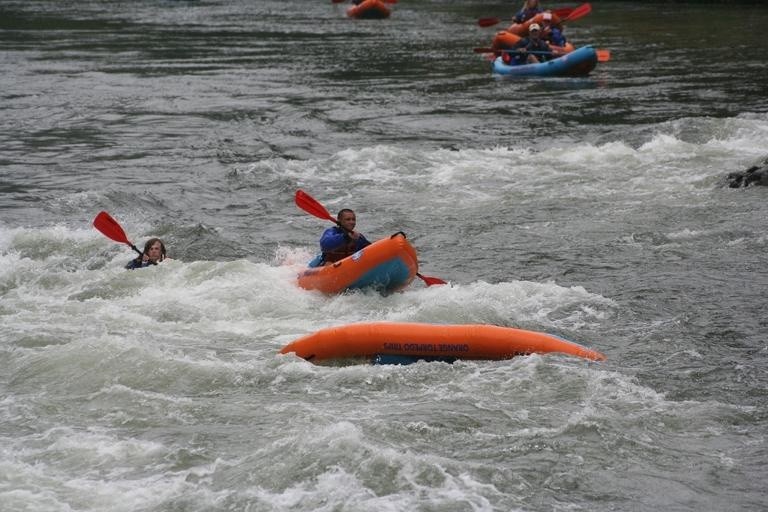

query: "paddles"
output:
93 211 156 265
479 16 512 27
296 190 370 245
554 4 590 21
473 44 610 63
415 271 447 285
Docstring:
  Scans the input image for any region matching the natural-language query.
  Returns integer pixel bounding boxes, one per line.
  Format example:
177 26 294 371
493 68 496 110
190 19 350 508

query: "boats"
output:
506 11 564 38
493 30 575 56
347 0 390 20
493 43 598 77
297 231 418 294
280 323 606 367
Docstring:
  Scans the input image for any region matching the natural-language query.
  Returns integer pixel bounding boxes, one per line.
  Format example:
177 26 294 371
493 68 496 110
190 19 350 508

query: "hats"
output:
543 13 552 21
529 23 541 32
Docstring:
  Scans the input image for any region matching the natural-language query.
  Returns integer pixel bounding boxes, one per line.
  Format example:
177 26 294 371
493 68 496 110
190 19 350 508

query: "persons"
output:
319 209 372 266
509 0 566 64
125 238 165 268
